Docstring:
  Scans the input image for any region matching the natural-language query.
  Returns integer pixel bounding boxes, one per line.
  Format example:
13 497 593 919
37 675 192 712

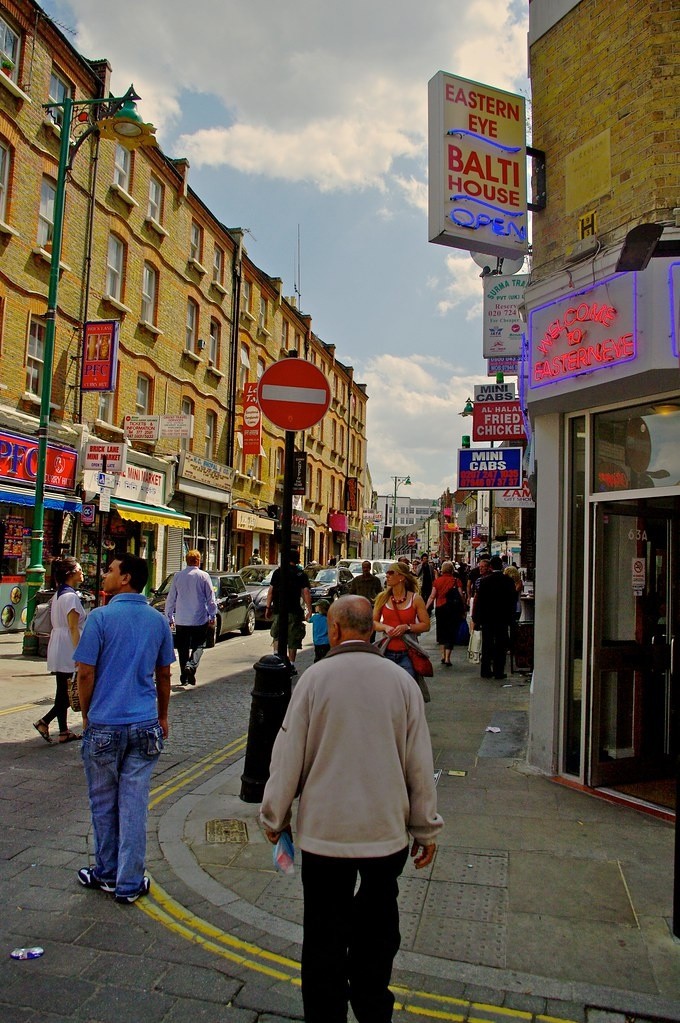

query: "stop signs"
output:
256 357 332 431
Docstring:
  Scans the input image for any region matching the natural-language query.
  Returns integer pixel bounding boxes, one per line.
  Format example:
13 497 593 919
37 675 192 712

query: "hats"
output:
311 599 330 611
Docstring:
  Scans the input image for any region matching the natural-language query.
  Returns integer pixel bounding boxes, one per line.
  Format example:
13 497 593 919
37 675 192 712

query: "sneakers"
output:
114 876 150 903
79 867 116 891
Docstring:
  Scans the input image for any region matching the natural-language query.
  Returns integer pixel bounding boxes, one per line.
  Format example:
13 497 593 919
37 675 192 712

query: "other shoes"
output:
482 672 490 679
180 674 188 685
495 674 507 679
445 662 452 666
441 659 445 663
185 664 195 685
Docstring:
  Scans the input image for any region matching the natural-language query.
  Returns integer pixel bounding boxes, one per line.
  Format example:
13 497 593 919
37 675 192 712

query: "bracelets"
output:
266 607 269 609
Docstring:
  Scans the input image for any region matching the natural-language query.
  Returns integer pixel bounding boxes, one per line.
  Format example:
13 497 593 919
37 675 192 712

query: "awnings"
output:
97 495 191 529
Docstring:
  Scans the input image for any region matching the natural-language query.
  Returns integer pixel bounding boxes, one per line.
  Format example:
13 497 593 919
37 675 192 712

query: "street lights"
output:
390 474 413 560
21 84 148 659
432 495 445 558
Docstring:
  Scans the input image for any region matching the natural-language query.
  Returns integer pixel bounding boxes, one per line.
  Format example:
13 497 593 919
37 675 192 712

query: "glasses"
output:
385 571 396 575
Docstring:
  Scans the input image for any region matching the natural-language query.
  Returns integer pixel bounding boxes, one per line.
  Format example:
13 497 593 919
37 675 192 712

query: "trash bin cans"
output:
37 591 54 657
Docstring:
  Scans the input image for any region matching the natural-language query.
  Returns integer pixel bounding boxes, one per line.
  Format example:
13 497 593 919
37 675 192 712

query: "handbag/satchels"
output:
407 647 433 677
31 599 53 638
467 623 483 664
445 577 464 615
67 672 84 712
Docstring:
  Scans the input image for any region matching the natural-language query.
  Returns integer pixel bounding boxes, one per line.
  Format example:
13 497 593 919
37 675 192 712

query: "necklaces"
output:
391 591 407 604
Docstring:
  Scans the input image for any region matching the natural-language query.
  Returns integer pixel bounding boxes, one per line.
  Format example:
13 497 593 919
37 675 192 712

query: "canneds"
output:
10 947 44 960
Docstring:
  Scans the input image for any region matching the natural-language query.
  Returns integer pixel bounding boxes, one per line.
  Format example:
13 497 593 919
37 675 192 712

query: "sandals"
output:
58 733 82 743
33 719 52 743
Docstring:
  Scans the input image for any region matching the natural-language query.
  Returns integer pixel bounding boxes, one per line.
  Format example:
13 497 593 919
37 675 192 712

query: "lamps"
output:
252 508 276 518
615 220 677 271
479 266 499 278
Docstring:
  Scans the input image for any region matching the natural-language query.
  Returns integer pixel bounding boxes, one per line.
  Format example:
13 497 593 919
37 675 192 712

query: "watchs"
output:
407 624 411 633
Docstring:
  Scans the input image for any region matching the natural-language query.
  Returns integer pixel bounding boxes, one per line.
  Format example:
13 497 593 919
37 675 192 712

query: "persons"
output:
251 549 261 565
472 557 517 679
330 555 336 566
370 562 430 679
33 557 87 744
165 550 217 685
306 599 330 663
351 561 382 644
71 551 177 904
398 553 524 667
265 549 312 672
260 595 444 1023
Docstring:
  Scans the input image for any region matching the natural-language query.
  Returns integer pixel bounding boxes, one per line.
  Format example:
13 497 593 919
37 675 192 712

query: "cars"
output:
233 565 281 629
148 571 258 649
334 554 460 592
303 564 354 605
